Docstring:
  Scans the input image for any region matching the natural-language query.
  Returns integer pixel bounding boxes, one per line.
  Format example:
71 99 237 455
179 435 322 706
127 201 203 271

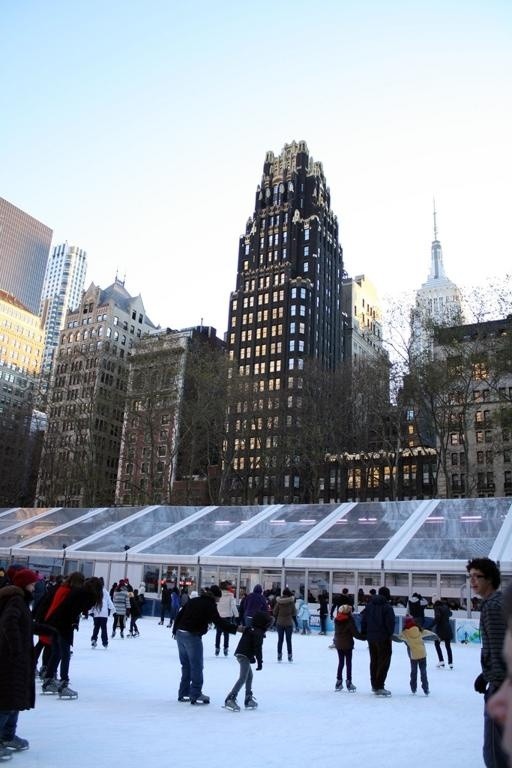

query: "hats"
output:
210 585 222 597
403 614 416 628
432 594 440 603
13 569 36 587
339 605 352 614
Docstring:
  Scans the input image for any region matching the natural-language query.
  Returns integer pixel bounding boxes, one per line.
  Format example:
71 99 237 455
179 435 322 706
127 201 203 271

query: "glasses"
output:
467 574 485 580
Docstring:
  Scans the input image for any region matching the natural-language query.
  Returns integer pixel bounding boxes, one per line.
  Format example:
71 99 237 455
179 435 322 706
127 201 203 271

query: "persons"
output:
161 580 299 712
300 586 458 698
83 578 146 649
31 570 103 699
466 558 511 767
1 563 43 759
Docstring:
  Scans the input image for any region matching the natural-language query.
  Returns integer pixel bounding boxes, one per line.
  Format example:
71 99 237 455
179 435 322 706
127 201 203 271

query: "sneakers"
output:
328 643 336 648
335 662 453 695
179 647 292 710
91 630 139 647
1 665 77 759
